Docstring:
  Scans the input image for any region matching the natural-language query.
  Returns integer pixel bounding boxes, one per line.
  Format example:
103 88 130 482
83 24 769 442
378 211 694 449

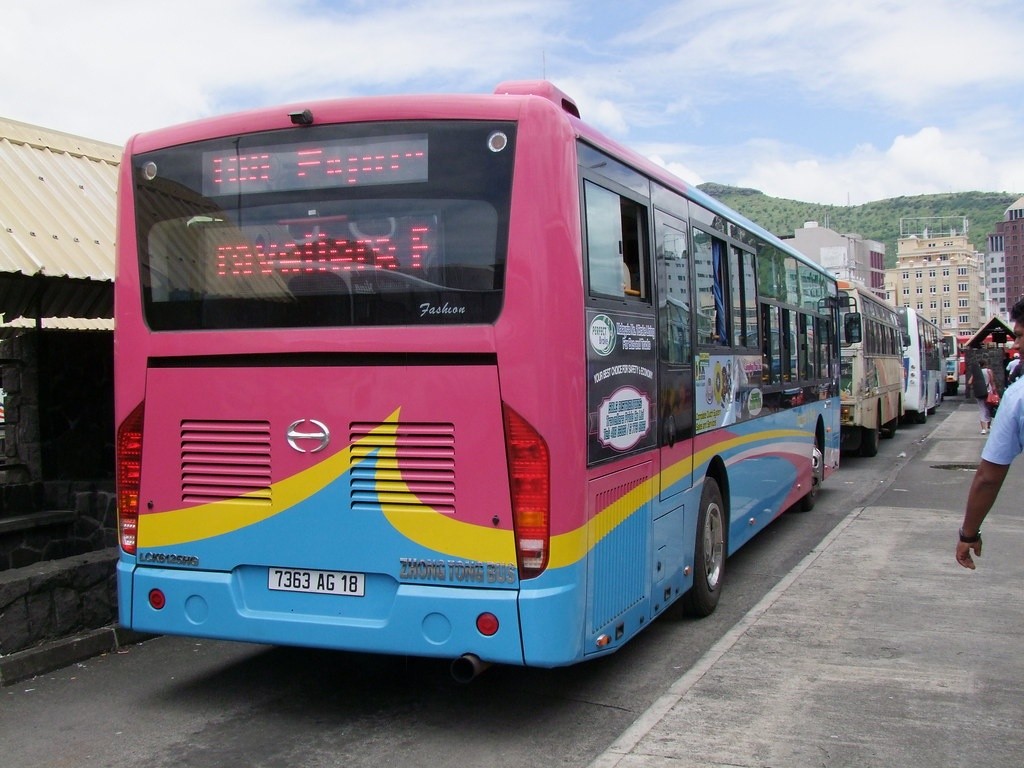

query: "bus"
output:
939 335 960 396
894 305 946 424
951 333 1020 377
834 279 913 456
118 79 863 693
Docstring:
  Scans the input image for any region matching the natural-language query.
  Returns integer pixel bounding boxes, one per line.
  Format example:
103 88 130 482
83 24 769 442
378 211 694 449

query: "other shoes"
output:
981 428 992 434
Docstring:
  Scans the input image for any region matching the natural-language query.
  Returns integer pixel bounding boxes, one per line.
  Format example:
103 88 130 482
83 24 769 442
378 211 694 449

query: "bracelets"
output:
958 528 981 543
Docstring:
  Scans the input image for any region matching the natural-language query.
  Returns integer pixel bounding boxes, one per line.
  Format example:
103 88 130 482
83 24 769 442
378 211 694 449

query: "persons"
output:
1003 353 1012 388
1006 353 1020 382
967 360 998 434
955 297 1024 570
1009 349 1024 381
